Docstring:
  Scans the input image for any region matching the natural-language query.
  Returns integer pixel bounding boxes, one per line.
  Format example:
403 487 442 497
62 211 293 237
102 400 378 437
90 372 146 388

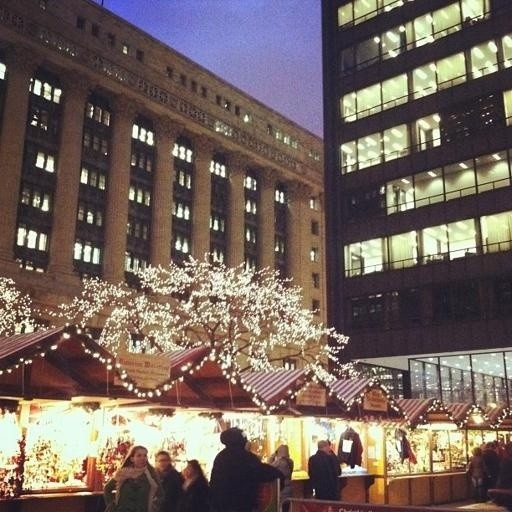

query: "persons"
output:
210 425 285 511
153 451 182 512
268 443 293 512
306 439 340 500
101 444 162 512
464 438 512 500
175 458 210 512
